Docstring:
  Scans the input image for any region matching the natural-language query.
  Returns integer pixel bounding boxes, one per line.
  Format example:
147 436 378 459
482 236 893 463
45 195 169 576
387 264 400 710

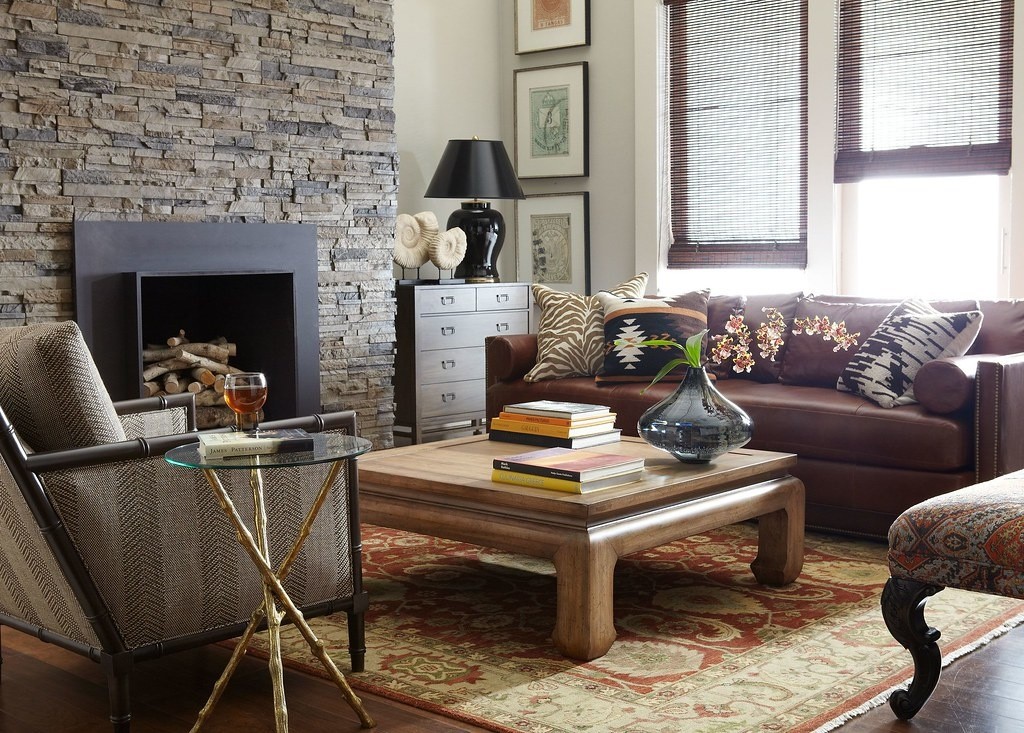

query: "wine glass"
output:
224 373 269 436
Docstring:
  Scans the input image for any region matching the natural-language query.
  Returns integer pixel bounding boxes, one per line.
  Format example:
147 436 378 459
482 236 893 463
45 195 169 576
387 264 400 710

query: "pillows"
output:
523 270 651 385
594 287 717 383
835 295 986 408
0 319 127 453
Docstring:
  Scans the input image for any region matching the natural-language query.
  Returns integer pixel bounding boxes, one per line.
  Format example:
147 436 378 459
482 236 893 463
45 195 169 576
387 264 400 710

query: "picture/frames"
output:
512 61 590 179
513 191 592 304
513 0 593 56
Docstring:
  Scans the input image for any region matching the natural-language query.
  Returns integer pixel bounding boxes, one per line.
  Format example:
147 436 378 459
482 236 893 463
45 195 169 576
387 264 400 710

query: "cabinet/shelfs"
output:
393 281 535 446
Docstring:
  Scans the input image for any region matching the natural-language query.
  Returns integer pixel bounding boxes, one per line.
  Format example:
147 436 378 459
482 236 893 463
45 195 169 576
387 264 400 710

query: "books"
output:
492 446 645 495
198 428 313 459
489 400 623 450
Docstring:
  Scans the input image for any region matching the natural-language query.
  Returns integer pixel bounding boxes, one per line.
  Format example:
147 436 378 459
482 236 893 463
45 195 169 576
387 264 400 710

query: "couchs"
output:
486 294 1024 544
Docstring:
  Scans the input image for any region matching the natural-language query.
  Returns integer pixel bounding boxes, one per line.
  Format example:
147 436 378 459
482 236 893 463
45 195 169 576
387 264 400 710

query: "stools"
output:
880 468 1024 721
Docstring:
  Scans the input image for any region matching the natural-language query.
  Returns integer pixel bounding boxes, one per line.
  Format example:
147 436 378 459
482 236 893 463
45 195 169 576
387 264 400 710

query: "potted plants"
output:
615 326 757 463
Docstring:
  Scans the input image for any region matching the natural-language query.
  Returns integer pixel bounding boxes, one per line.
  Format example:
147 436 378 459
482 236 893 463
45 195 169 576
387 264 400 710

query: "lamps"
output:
422 134 526 282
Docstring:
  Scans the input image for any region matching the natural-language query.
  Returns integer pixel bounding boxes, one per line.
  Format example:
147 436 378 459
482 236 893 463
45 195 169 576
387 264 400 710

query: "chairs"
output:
0 392 368 733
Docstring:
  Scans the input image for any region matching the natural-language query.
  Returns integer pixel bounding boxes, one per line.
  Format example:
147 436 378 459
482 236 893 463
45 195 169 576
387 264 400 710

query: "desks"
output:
162 432 374 733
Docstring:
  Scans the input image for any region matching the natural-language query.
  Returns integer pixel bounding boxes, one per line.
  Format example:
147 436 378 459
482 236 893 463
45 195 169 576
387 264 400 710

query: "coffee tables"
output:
355 431 808 661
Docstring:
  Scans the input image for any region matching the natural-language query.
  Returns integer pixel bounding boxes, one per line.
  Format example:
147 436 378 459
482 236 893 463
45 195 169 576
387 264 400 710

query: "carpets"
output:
221 518 1024 733
